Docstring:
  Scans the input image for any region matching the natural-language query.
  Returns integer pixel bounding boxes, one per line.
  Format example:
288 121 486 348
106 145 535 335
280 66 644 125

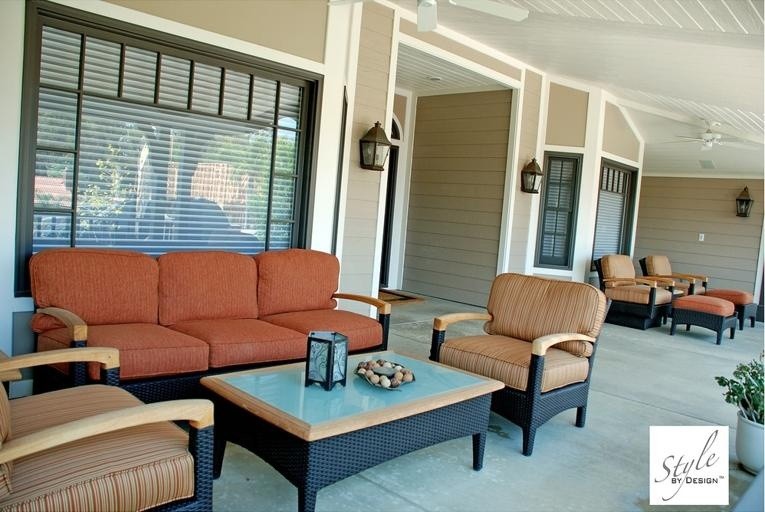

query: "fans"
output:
657 117 759 154
328 1 530 33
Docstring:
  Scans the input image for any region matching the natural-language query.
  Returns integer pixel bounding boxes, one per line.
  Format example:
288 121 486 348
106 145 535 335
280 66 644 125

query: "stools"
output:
666 294 736 345
690 286 757 330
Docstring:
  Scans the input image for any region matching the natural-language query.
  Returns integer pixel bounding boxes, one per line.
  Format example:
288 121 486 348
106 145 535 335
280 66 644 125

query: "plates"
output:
353 360 416 391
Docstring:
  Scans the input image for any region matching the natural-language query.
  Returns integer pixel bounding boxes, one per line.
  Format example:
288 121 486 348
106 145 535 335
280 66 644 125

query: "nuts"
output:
357 359 413 388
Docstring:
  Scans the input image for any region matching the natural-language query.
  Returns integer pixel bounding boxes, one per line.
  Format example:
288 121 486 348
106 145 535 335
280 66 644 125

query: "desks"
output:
199 349 506 511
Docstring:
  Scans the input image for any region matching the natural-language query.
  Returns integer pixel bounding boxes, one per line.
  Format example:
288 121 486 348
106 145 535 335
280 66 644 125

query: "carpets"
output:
378 287 426 307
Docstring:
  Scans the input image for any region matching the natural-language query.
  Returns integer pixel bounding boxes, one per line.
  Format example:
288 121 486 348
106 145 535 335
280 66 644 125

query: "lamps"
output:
359 120 391 173
519 159 544 194
734 184 754 219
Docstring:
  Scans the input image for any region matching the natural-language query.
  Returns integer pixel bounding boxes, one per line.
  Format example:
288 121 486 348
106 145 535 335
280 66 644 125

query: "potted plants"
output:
715 360 764 478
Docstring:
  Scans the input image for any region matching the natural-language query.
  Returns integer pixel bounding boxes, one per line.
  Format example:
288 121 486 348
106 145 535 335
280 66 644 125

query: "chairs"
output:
594 255 672 331
430 269 612 458
0 336 219 512
640 253 708 330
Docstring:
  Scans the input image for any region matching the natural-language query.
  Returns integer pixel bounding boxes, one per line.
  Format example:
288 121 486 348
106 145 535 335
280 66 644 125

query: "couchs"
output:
23 250 390 403
